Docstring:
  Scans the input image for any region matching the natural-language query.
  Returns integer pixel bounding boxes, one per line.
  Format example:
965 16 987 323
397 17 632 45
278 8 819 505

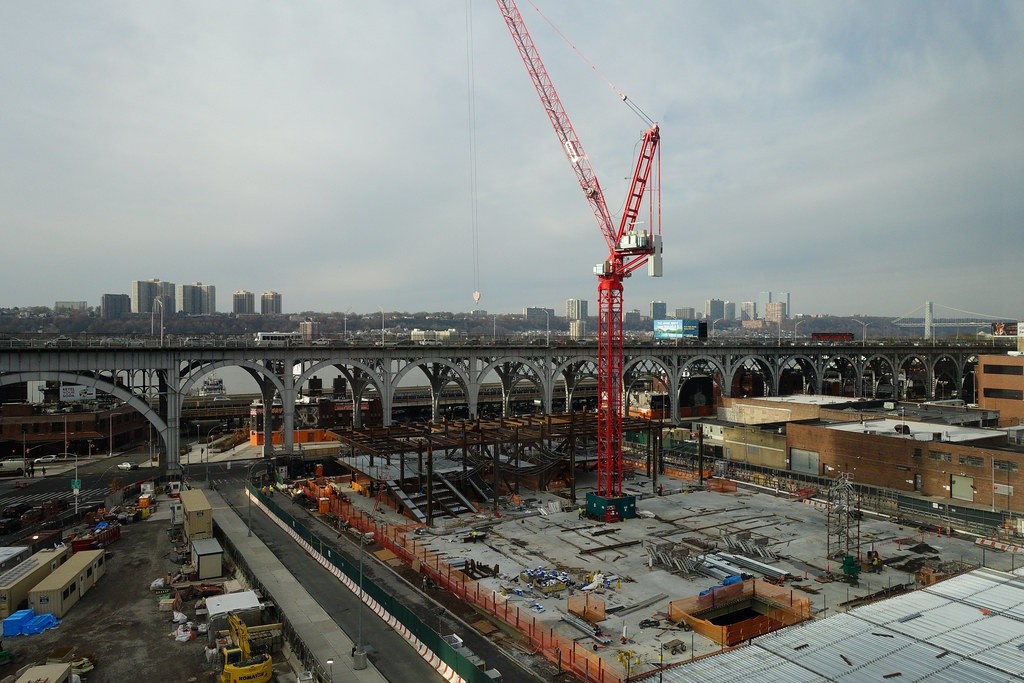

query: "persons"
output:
872 554 877 573
30 467 35 478
269 485 274 497
30 460 34 469
262 486 267 496
423 576 427 591
42 467 45 475
26 468 31 477
578 506 583 520
470 529 477 544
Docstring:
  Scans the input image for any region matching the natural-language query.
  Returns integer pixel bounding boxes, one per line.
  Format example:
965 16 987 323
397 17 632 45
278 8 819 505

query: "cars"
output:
0 499 70 536
0 332 1019 352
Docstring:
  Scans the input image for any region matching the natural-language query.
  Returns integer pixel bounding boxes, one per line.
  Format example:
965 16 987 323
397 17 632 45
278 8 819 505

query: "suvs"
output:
34 454 59 463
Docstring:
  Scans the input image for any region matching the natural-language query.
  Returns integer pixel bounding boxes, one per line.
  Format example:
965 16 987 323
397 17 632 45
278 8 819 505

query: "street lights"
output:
149 296 165 345
852 318 865 348
110 412 123 456
864 322 872 340
58 452 78 514
204 423 229 490
712 317 724 339
794 320 806 341
353 531 377 669
541 307 550 346
246 455 277 538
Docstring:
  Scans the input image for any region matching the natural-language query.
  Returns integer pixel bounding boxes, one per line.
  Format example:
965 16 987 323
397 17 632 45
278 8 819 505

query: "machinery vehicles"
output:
214 611 273 683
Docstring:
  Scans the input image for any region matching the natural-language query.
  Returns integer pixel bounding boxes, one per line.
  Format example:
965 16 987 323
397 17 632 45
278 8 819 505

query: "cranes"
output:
462 0 665 524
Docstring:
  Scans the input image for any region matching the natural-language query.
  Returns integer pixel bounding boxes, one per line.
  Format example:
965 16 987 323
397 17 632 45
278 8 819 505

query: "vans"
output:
0 458 30 476
